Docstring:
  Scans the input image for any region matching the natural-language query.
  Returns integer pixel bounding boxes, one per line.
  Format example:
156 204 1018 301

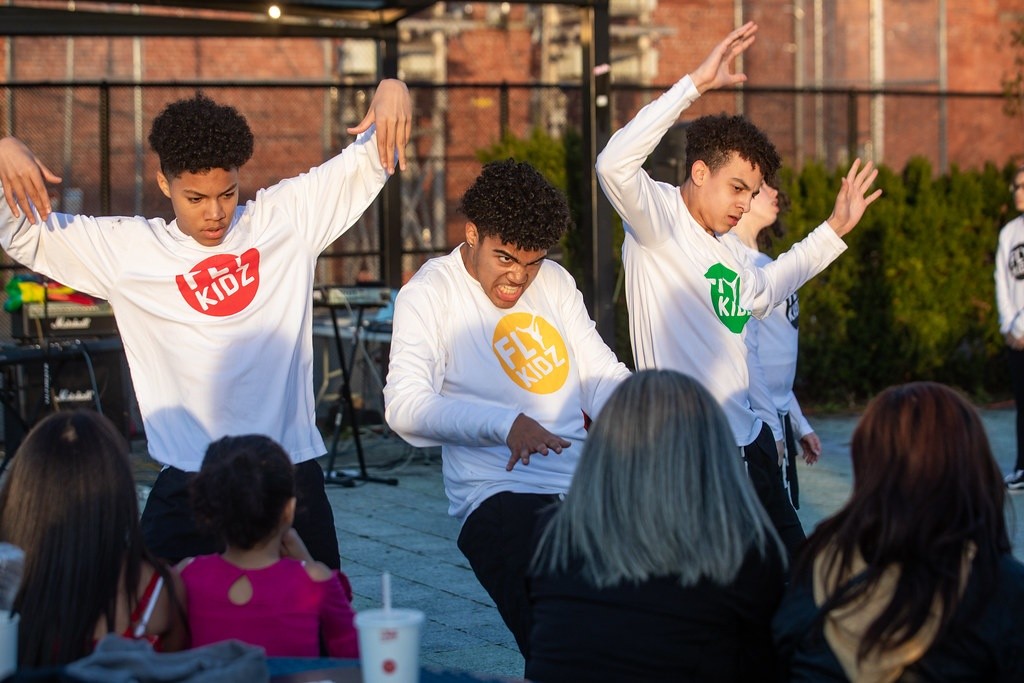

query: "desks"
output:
312 311 440 466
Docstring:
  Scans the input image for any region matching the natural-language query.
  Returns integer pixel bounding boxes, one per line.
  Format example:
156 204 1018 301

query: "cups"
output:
354 608 425 683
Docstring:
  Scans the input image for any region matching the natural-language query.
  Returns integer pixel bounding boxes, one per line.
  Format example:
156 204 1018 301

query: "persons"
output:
0 406 173 683
381 156 638 683
0 78 414 604
739 170 822 544
171 433 360 683
522 368 791 683
792 381 1024 683
992 167 1024 497
593 18 884 603
